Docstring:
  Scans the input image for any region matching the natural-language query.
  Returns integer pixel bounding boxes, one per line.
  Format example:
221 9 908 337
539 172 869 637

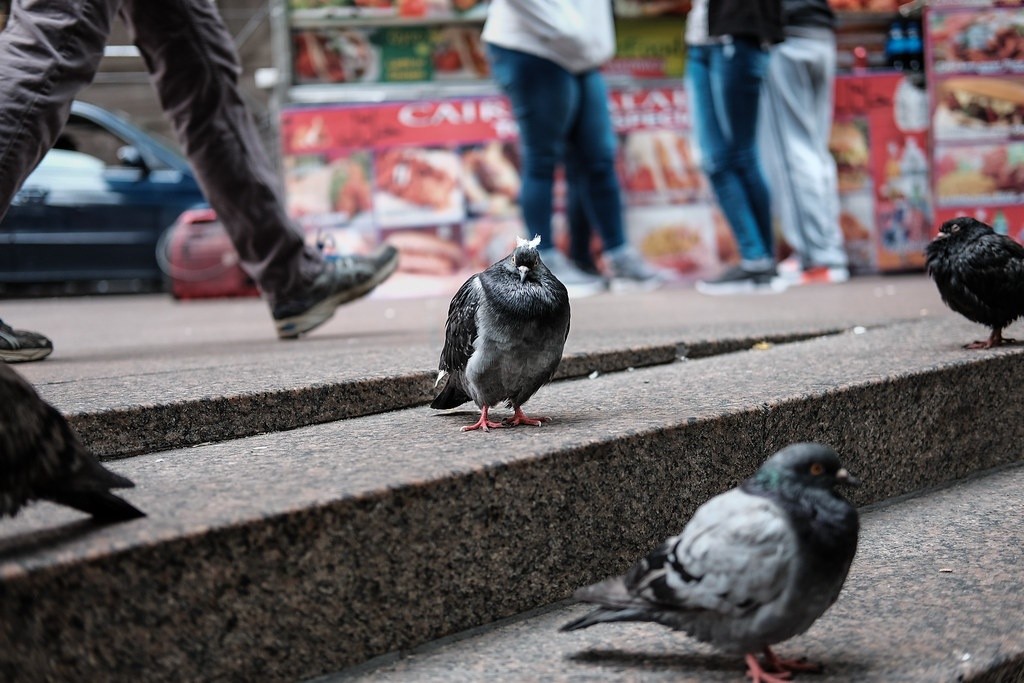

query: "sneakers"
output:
260 246 399 339
0 319 52 363
538 240 850 299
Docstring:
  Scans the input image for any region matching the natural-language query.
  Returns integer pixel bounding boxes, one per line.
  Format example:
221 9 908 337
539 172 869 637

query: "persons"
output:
684 0 777 296
757 0 847 286
481 0 677 289
0 0 396 361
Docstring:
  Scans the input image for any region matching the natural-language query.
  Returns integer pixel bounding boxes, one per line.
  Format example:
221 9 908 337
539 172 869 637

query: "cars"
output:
1 100 211 283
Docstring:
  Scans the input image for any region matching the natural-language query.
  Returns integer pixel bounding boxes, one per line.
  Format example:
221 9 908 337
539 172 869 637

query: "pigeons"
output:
427 233 572 435
924 216 1023 351
0 354 147 524
556 444 863 683
